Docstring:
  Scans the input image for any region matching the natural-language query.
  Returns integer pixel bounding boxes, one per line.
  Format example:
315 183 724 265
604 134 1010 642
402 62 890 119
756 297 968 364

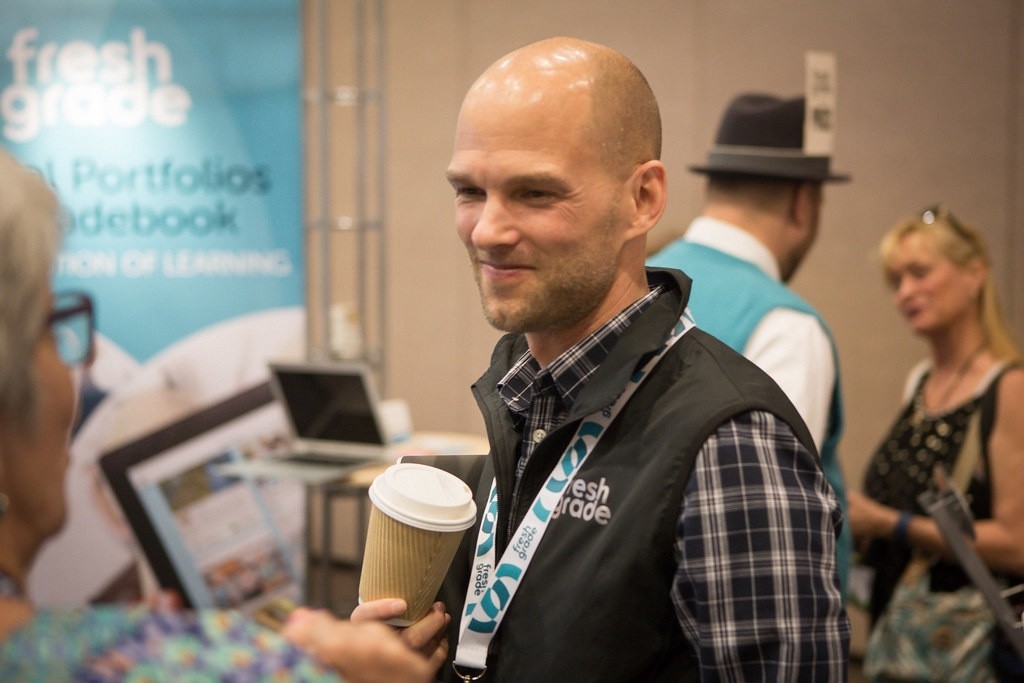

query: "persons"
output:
645 93 847 616
0 146 434 683
349 37 851 683
853 204 1024 683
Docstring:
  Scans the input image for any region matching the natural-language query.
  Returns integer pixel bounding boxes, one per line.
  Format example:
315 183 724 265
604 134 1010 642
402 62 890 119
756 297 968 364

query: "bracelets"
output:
889 509 913 545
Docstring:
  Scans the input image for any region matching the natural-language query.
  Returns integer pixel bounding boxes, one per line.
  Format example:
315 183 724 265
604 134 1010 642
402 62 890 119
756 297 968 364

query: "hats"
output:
686 93 852 181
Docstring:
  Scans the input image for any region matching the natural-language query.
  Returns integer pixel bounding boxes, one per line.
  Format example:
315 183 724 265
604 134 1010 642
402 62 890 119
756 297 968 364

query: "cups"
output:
359 463 477 626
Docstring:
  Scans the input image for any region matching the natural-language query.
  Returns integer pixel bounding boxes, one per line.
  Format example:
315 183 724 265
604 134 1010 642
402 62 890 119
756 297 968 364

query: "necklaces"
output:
912 340 988 427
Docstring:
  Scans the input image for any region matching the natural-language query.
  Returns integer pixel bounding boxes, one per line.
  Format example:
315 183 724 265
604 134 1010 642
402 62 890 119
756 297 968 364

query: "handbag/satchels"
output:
862 489 1024 683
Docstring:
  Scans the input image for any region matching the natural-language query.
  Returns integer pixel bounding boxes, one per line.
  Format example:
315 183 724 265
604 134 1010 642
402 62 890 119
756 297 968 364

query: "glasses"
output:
894 203 979 250
0 291 95 369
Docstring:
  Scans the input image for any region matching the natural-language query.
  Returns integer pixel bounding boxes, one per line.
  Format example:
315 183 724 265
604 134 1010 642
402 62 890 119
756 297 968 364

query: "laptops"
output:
269 362 385 448
